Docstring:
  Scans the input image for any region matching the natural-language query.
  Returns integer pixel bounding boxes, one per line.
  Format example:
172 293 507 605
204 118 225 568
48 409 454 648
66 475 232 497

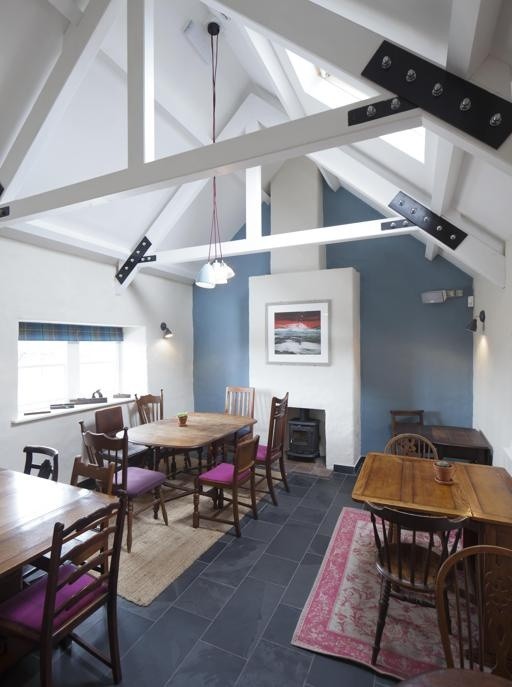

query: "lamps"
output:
195 22 235 289
465 310 485 332
160 322 174 338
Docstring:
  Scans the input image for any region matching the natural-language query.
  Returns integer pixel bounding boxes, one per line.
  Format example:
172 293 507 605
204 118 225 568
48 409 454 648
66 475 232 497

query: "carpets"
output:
291 505 492 681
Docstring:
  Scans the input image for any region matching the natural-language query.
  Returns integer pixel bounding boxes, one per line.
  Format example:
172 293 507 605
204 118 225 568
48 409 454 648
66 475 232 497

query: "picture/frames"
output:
265 299 331 366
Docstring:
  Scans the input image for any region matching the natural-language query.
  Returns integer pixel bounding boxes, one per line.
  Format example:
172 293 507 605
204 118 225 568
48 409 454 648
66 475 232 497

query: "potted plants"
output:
433 460 457 486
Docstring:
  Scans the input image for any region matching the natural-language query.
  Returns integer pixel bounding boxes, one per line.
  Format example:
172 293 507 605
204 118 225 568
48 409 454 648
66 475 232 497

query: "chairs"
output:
390 409 424 425
394 545 512 687
363 499 470 665
384 433 450 558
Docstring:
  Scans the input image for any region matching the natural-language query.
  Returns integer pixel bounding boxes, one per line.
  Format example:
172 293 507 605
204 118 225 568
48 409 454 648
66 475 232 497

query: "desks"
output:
390 423 493 466
352 452 512 668
